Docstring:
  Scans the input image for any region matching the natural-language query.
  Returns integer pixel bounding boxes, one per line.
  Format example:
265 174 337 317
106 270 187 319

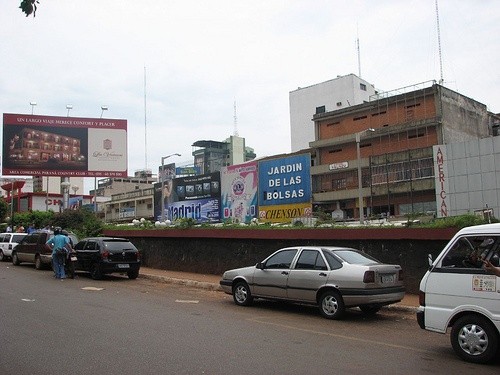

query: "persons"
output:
45 227 76 281
6 219 54 234
480 259 500 277
62 230 74 279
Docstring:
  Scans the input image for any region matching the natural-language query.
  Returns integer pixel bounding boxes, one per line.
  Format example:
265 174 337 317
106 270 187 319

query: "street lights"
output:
60 182 71 211
355 129 375 225
162 153 182 219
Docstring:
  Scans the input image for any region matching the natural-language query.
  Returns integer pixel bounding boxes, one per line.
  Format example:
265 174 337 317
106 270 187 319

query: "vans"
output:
418 224 500 361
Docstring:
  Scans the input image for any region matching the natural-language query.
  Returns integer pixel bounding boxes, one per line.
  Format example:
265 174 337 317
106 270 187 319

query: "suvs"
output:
12 230 80 269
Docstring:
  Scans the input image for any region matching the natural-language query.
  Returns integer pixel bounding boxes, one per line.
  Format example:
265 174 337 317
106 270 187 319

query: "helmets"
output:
61 230 68 237
54 227 62 235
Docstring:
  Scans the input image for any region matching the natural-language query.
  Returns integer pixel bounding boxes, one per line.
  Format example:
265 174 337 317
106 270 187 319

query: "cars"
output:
218 245 405 319
0 232 29 261
68 236 141 279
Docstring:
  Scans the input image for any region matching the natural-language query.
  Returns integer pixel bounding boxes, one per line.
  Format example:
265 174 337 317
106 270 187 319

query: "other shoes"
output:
54 277 65 281
